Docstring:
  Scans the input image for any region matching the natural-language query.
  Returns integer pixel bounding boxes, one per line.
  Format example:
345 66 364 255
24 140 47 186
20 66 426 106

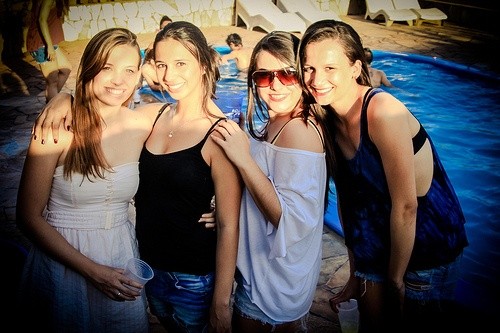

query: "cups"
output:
121 259 154 297
213 91 243 126
336 299 360 333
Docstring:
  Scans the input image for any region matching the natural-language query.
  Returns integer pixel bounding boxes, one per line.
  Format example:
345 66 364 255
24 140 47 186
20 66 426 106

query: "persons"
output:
220 33 254 73
15 27 216 333
139 15 173 91
1 0 72 104
31 22 243 333
360 48 392 89
210 31 326 333
294 19 468 333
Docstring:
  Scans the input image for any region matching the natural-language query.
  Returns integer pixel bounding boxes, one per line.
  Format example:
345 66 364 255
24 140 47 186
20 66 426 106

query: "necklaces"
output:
169 99 212 138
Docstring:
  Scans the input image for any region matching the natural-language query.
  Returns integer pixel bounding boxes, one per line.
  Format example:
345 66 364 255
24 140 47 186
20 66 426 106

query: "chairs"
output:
236 0 307 36
275 0 341 29
364 0 418 28
391 0 448 28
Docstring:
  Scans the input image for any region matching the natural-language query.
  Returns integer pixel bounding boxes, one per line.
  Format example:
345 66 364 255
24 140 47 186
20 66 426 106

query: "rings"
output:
118 292 121 296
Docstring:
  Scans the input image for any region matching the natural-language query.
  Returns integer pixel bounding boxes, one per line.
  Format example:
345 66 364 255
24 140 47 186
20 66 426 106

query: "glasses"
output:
251 69 301 87
